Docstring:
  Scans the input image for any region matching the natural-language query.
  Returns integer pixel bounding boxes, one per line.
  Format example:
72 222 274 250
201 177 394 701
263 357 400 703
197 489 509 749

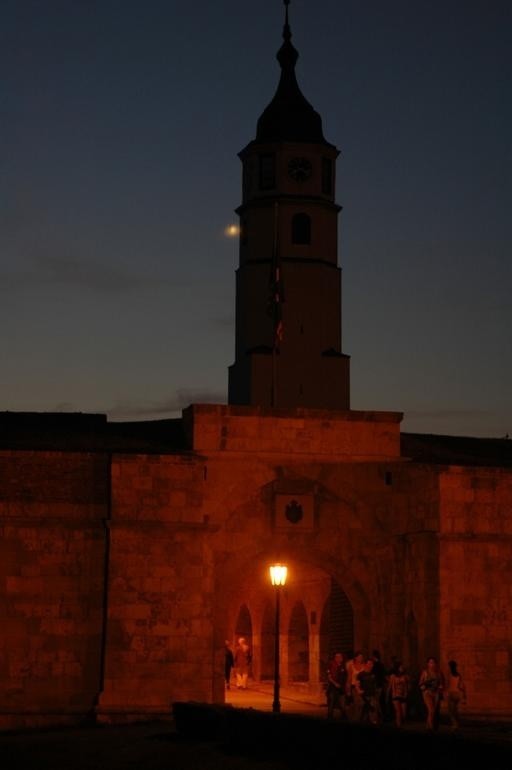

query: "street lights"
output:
270 559 290 714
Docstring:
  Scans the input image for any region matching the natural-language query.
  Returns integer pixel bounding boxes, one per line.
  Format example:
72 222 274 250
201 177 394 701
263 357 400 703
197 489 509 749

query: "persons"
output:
324 645 415 729
222 640 235 690
442 660 469 732
418 655 447 730
232 637 250 689
